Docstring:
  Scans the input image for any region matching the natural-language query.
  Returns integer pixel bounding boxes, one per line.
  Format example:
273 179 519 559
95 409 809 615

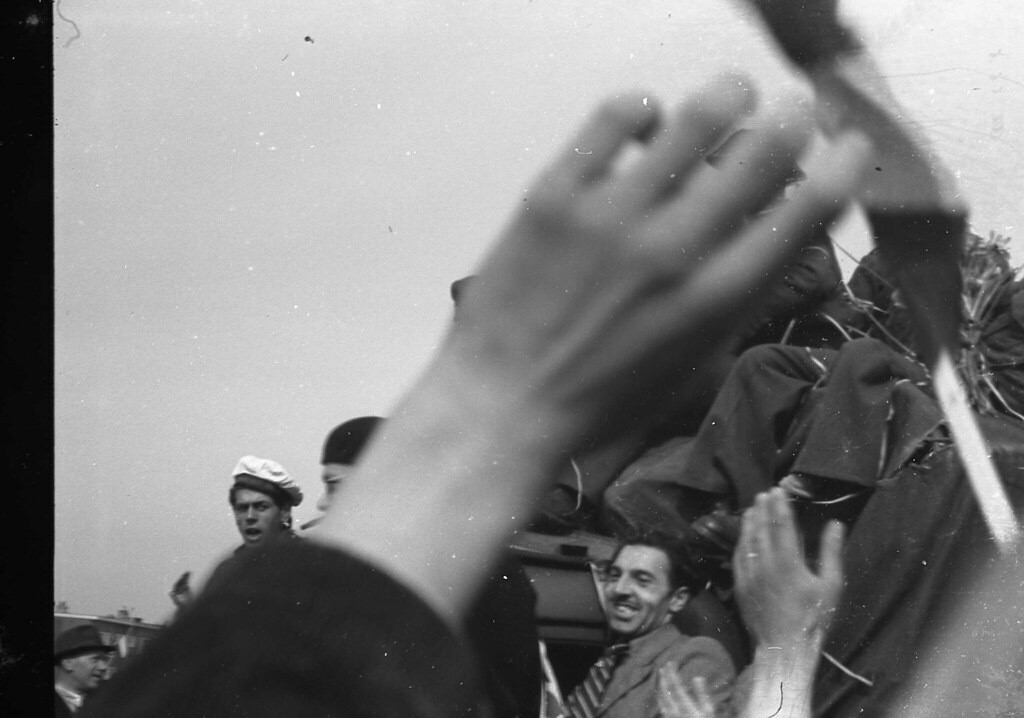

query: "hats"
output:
54 624 118 665
318 417 388 466
232 455 306 511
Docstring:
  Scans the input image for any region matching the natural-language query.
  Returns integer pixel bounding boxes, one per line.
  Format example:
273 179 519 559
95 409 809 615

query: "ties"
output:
563 642 621 718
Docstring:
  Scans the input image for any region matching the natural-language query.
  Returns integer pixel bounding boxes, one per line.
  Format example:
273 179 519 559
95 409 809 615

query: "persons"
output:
56 71 1024 718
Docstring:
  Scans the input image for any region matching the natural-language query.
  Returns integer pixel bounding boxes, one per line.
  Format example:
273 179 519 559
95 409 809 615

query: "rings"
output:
746 552 760 559
